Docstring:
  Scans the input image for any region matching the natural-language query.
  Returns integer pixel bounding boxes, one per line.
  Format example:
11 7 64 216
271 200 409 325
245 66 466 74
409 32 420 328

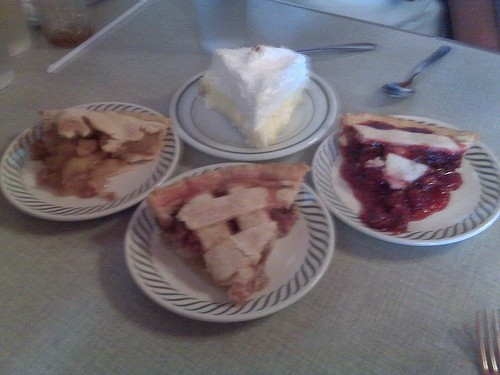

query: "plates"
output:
309 112 500 247
0 100 179 223
168 65 336 162
121 161 336 325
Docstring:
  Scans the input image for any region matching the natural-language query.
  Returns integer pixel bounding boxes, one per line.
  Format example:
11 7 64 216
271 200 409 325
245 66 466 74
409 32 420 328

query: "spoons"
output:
383 45 455 100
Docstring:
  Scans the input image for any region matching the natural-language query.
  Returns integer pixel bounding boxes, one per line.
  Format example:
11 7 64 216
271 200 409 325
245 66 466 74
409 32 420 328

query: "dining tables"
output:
1 0 500 375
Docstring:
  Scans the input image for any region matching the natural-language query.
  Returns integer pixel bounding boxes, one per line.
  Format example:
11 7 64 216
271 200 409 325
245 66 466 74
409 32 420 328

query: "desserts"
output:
198 42 309 147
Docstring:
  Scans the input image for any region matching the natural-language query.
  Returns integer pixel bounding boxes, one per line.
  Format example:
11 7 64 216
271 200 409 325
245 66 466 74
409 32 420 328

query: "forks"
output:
474 306 499 373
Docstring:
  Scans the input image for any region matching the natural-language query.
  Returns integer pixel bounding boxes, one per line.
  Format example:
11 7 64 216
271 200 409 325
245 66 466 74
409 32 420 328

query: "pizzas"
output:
147 160 310 303
342 111 480 236
30 106 170 198
